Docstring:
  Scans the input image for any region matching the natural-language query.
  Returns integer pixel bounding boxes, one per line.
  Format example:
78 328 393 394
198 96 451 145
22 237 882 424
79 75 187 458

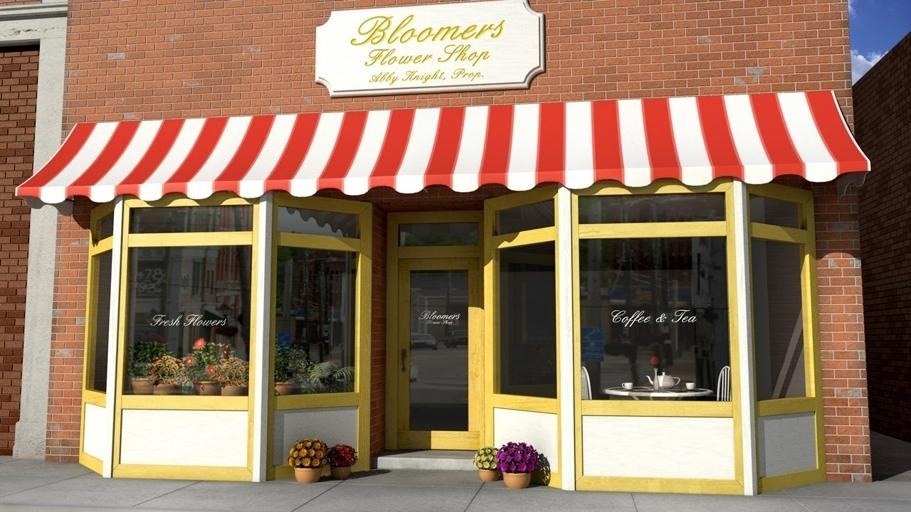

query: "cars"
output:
442 329 467 349
411 331 439 351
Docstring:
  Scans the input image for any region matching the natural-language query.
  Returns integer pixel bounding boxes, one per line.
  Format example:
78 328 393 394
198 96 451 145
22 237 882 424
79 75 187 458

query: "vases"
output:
479 470 500 481
503 472 532 489
294 466 324 483
330 467 353 478
155 379 247 398
653 366 660 390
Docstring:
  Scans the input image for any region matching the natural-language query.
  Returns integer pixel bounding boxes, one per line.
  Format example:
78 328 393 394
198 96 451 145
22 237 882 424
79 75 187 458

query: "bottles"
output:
654 368 660 391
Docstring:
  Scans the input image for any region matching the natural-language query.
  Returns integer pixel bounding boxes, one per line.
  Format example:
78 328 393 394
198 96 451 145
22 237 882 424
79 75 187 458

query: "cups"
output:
621 382 635 390
685 382 696 390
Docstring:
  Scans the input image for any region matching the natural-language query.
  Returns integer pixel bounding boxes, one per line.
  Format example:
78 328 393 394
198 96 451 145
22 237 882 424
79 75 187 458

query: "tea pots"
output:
646 371 682 389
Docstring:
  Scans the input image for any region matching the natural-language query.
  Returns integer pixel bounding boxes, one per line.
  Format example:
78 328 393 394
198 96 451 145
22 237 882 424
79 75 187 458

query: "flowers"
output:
472 448 504 470
147 338 252 393
288 437 327 470
650 356 660 367
496 440 538 474
328 444 356 468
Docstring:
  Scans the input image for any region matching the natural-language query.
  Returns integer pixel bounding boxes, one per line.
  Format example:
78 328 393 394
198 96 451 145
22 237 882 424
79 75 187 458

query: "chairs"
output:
581 365 595 400
716 364 733 401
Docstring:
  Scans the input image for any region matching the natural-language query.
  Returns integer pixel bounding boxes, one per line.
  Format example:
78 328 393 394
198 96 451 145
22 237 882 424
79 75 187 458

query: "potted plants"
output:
274 338 304 394
127 342 167 394
